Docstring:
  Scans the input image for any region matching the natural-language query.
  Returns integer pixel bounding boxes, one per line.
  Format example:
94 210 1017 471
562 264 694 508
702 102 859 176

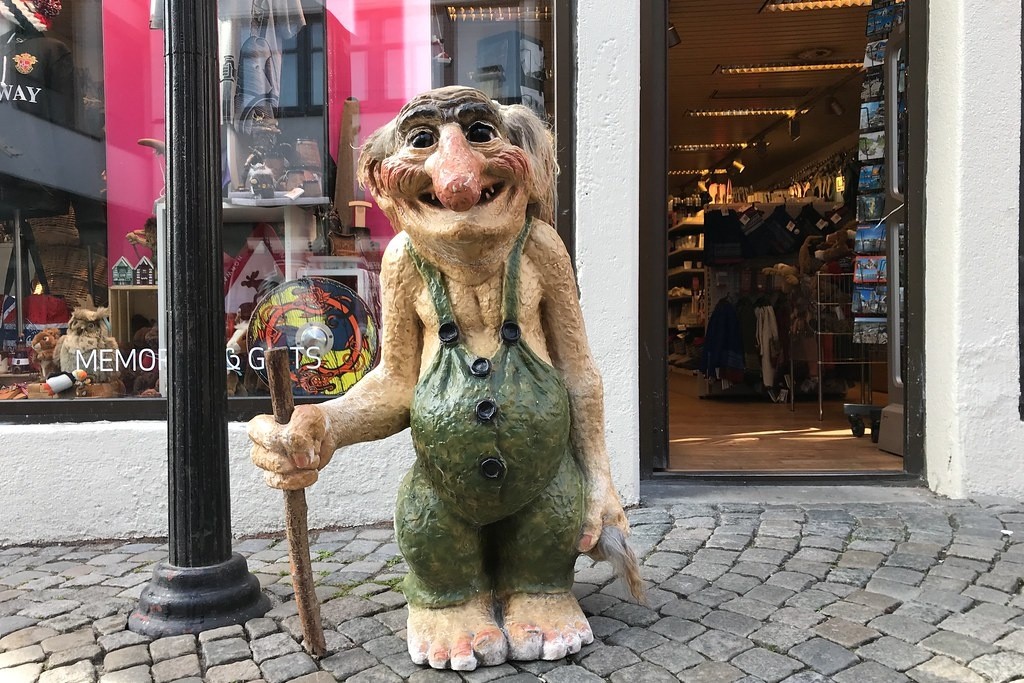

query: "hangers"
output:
754 141 859 193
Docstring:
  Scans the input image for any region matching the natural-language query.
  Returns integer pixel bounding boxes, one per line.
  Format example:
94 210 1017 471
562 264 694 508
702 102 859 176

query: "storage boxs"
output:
878 400 904 456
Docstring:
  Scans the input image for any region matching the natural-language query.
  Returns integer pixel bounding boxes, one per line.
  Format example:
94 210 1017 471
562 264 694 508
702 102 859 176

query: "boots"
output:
666 342 700 368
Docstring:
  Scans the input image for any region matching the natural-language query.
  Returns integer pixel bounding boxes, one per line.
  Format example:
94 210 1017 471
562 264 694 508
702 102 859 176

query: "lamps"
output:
682 108 808 118
668 142 771 153
713 57 864 75
787 119 800 141
757 139 766 152
668 168 727 175
758 0 872 13
732 160 745 173
829 98 843 115
667 22 681 48
446 4 553 23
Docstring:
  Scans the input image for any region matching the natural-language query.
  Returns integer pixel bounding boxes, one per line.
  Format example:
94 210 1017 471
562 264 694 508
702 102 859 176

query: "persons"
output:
247 86 645 672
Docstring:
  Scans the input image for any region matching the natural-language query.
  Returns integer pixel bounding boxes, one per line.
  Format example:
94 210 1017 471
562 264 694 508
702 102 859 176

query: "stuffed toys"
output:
761 220 853 336
31 306 162 398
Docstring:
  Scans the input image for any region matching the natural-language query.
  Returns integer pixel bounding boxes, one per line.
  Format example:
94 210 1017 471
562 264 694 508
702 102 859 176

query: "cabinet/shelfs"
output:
221 195 381 328
667 203 886 420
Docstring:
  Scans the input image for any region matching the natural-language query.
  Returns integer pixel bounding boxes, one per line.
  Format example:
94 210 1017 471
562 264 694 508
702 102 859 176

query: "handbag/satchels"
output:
0 222 68 353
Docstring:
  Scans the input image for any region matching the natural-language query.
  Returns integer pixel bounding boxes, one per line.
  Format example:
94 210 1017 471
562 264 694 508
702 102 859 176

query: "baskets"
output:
27 203 80 246
29 242 108 311
